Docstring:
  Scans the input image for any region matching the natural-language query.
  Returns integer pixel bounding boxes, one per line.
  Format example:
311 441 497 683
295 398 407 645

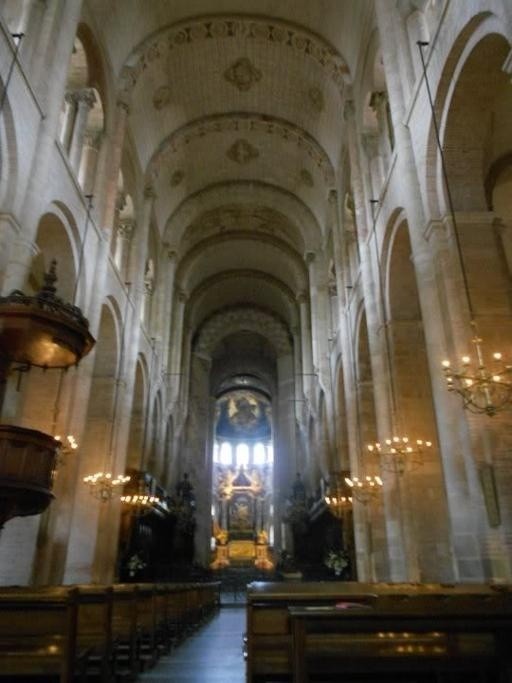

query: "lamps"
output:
51 198 91 476
119 342 160 515
343 290 384 504
414 40 511 418
367 200 433 476
84 283 131 502
324 343 352 516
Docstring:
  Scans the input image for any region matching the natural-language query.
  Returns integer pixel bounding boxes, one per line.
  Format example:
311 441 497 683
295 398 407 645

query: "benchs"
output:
0 580 224 683
243 580 512 683
